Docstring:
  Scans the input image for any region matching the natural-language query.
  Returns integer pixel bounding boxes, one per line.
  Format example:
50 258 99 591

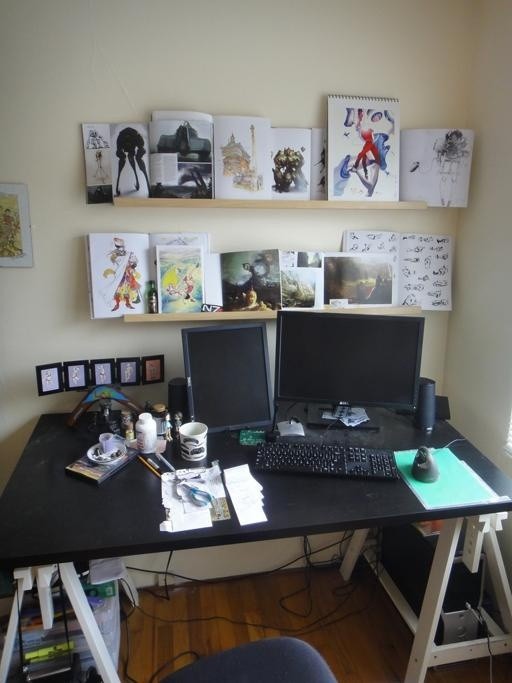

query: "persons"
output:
45 369 54 389
70 365 80 386
96 363 107 384
123 362 133 382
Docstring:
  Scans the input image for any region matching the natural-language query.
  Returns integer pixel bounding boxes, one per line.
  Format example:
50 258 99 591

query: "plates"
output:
87 442 127 465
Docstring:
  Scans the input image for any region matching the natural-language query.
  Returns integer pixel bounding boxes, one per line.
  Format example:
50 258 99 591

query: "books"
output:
0 559 97 683
394 447 492 509
65 433 141 486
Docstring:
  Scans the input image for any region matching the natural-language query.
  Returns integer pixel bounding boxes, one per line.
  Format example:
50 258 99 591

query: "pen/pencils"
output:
154 451 176 471
145 456 160 471
137 455 161 479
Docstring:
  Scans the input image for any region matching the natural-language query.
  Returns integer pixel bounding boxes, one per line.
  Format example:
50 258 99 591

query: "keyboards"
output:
251 441 399 480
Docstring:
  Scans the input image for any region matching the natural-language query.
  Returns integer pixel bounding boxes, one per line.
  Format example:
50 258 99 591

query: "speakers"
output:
413 377 435 435
168 376 190 428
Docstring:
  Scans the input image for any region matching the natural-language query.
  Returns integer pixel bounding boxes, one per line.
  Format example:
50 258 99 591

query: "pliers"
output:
183 483 212 506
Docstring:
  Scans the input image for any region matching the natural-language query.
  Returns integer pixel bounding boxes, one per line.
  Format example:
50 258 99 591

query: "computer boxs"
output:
379 521 487 672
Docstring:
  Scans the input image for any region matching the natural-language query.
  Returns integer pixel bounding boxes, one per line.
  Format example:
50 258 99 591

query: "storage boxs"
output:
11 574 122 671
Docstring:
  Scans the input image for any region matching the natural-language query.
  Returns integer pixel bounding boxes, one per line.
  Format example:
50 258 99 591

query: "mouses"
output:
413 447 440 483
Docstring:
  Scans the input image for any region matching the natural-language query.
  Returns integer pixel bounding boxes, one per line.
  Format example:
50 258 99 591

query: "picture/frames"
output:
0 181 33 267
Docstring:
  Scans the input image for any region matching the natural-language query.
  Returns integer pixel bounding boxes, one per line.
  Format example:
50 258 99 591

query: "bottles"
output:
118 400 172 453
147 279 158 313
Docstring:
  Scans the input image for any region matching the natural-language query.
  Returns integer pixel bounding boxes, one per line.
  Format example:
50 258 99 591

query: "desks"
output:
1 394 510 682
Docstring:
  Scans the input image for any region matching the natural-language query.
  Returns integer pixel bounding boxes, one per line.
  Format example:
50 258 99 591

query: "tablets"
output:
181 322 275 434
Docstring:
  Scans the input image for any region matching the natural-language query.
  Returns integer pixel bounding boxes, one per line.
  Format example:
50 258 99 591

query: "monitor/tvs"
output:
275 311 425 431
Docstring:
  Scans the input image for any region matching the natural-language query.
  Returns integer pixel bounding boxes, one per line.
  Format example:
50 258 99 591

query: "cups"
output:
178 420 208 461
98 433 114 453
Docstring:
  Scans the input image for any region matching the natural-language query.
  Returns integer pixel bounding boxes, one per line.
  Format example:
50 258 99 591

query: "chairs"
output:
155 638 336 681
